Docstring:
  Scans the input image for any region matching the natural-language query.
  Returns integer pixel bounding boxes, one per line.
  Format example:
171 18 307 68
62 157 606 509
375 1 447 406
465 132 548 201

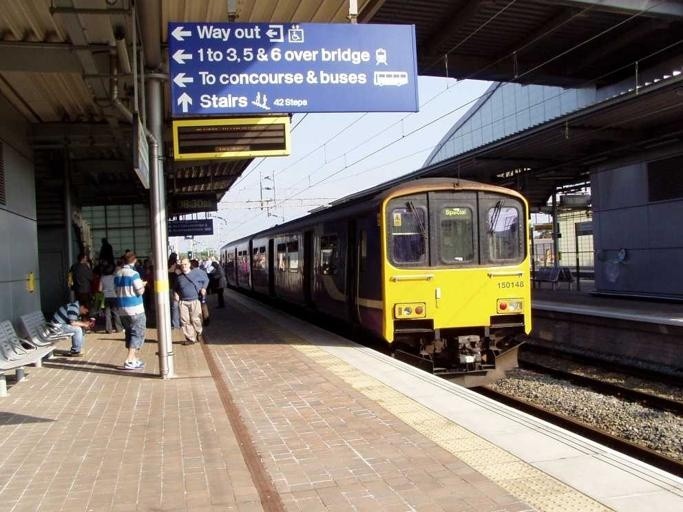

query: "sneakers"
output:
124 358 145 370
184 335 203 345
107 329 123 333
72 353 85 357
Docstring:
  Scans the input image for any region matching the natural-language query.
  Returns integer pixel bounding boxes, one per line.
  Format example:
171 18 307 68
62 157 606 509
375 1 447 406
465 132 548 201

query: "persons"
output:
210 262 226 307
221 251 292 289
50 238 210 369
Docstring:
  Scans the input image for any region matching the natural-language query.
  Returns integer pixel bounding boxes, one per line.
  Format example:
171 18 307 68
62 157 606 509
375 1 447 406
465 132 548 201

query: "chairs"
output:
0 307 74 396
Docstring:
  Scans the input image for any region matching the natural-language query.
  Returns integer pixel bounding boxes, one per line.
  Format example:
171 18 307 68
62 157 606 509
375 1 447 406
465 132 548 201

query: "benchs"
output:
530 264 575 291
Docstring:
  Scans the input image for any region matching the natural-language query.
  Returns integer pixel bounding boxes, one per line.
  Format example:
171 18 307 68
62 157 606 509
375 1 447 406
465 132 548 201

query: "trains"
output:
219 176 533 390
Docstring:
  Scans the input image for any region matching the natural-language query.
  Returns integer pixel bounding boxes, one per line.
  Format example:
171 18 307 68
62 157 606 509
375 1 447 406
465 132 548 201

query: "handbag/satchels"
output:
201 303 209 326
